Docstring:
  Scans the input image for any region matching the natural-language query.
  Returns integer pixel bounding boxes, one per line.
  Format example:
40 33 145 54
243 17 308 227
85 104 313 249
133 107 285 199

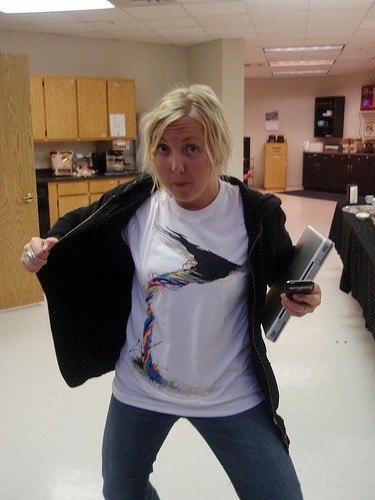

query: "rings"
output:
301 304 308 314
25 251 34 260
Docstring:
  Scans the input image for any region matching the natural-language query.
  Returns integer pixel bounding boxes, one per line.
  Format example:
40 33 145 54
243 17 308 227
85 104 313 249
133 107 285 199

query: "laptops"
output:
262 226 335 341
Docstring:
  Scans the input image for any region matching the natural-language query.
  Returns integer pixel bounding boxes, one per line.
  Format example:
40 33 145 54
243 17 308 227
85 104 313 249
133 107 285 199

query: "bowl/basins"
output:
356 212 370 221
317 120 325 127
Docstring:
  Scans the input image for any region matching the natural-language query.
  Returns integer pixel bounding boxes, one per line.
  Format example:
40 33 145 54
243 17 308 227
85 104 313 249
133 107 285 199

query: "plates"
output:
342 205 375 215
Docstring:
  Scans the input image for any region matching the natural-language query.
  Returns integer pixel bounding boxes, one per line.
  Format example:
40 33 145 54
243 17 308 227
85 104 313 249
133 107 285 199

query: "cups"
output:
372 198 375 205
365 195 374 205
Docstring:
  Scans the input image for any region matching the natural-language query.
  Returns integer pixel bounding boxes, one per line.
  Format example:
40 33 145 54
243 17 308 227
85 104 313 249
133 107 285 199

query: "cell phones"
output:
284 280 314 299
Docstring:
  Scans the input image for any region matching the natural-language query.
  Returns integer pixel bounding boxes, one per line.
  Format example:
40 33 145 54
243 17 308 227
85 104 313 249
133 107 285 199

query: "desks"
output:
302 152 375 198
329 201 375 342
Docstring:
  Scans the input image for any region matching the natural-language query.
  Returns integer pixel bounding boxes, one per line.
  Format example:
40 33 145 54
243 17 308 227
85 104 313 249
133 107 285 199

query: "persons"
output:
20 83 323 500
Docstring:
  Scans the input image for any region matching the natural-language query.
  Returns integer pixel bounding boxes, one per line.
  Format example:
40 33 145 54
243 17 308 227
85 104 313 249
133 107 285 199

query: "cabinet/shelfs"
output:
313 96 345 138
357 110 375 147
264 140 287 192
30 77 137 232
